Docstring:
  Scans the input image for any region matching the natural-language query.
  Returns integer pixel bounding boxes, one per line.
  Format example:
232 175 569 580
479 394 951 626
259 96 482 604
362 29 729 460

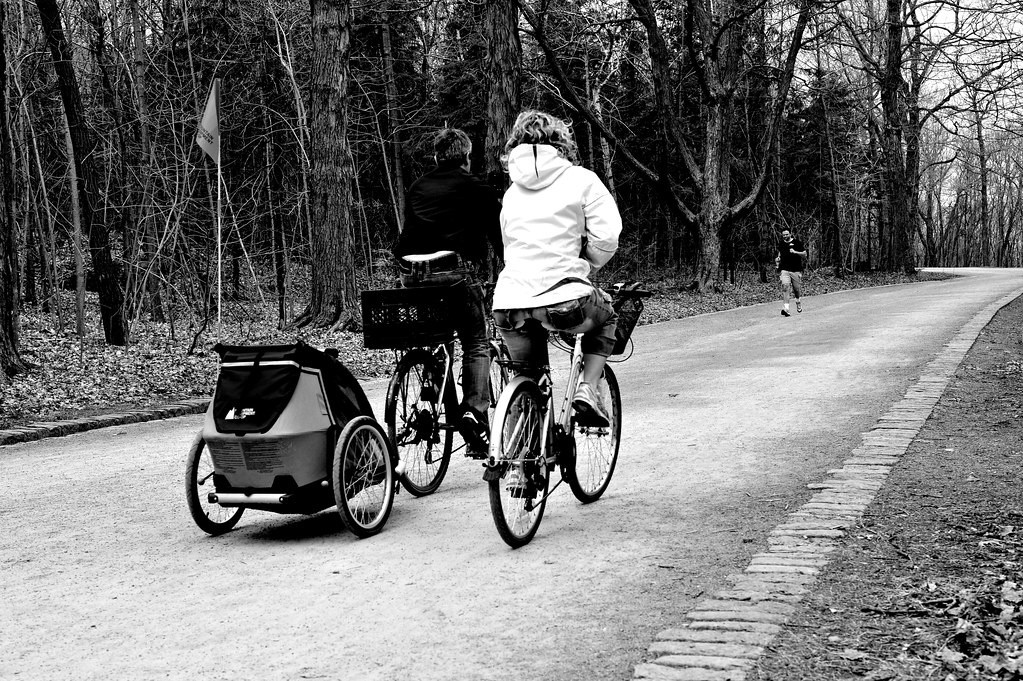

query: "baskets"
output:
560 292 642 355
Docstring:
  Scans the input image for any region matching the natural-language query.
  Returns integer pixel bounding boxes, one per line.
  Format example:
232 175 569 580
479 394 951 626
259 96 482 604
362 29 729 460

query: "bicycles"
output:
383 283 515 495
479 282 650 549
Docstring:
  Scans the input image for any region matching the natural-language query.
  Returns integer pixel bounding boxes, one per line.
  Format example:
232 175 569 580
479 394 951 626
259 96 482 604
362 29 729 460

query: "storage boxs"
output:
362 287 453 350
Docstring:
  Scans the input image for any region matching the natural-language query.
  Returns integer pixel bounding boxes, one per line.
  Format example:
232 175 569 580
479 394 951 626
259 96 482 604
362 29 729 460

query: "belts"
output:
397 262 453 276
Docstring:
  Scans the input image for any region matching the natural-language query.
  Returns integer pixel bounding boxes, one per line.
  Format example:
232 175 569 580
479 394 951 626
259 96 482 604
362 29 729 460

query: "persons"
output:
776 228 808 316
493 110 623 493
394 129 504 454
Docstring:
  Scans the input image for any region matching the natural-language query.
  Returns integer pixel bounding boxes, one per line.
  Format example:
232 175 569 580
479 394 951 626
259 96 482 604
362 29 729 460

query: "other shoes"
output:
796 302 803 314
782 310 791 318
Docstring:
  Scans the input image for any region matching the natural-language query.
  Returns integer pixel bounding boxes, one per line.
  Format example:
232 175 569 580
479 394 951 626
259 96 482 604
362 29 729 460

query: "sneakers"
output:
506 470 528 491
572 382 611 428
460 410 490 455
